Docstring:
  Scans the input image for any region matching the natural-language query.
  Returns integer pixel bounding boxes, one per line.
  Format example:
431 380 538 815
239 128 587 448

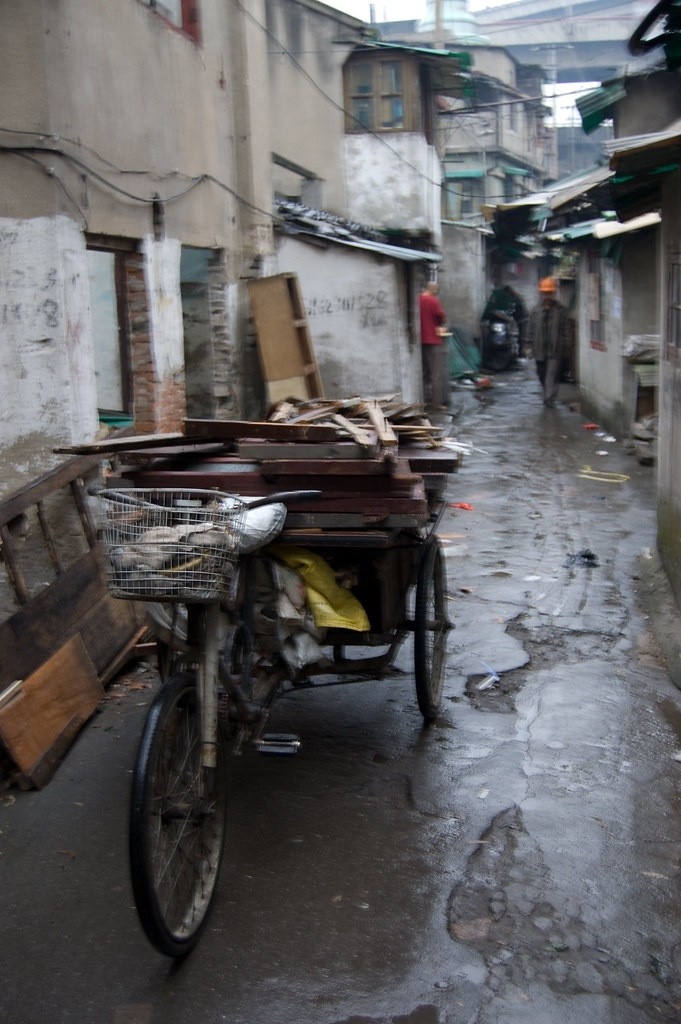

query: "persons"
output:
419 281 449 410
523 278 576 409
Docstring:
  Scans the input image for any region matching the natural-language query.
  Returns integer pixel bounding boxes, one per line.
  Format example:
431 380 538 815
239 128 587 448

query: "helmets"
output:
435 327 454 337
538 278 558 292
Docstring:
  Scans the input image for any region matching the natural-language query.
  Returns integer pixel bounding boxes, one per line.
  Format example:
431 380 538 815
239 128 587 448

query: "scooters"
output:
482 313 514 372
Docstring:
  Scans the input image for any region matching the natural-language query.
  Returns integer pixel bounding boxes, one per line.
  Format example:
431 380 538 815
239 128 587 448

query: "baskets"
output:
96 488 250 606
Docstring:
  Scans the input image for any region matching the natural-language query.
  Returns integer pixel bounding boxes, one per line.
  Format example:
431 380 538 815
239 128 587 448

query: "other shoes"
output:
544 401 557 409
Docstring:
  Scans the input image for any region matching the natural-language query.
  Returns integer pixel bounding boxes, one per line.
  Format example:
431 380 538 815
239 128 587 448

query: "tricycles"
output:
77 468 459 964
